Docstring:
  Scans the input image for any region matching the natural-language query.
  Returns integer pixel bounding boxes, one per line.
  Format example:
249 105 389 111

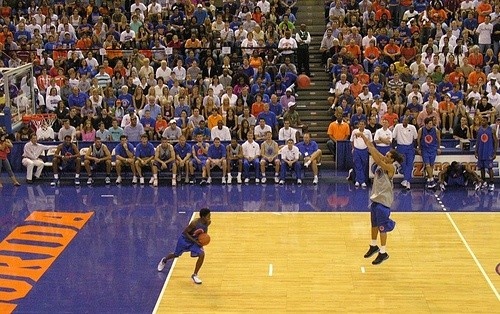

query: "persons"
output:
260 131 280 184
350 119 374 187
173 135 192 183
353 132 403 264
84 137 112 185
437 161 487 191
417 117 441 189
206 137 227 184
279 133 322 185
152 137 177 186
22 133 59 184
0 179 500 242
475 116 497 192
279 139 303 185
392 115 418 190
0 127 20 188
115 135 137 184
50 135 81 185
319 0 500 166
242 132 262 183
158 208 211 284
189 133 210 186
226 137 244 184
134 134 158 187
0 0 314 165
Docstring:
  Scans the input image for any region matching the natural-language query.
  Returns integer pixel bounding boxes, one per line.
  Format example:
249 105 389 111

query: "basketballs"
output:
198 233 210 245
297 75 310 88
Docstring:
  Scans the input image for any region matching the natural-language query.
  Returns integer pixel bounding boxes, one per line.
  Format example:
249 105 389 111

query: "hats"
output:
128 107 135 112
99 65 105 68
446 93 451 98
286 88 292 93
110 24 116 28
373 94 380 99
197 4 202 8
173 6 178 10
411 76 419 80
130 115 137 120
288 102 296 107
170 119 177 124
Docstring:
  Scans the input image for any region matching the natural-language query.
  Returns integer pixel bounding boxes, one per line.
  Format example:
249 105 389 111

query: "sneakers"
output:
140 177 144 183
74 178 80 184
274 176 279 182
116 176 122 184
372 252 389 265
327 88 335 94
297 178 302 184
313 177 318 184
222 177 226 184
261 178 266 183
50 178 60 185
132 176 137 183
199 178 206 187
87 177 95 185
149 177 154 183
364 245 380 258
191 275 202 284
355 181 359 186
105 177 110 183
206 177 212 184
190 174 196 184
158 256 167 272
279 180 283 185
361 183 367 186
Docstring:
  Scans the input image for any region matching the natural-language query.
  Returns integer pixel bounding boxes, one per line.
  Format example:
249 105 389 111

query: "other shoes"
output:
425 177 437 188
440 183 446 192
255 178 259 182
227 176 233 183
172 178 176 186
449 128 453 134
489 184 494 191
405 182 411 190
153 178 158 186
245 178 250 182
237 176 242 183
456 144 461 148
475 182 482 192
35 179 40 184
482 181 487 188
304 162 309 168
0 184 3 187
400 180 407 187
185 177 189 183
26 180 33 184
442 127 446 134
14 181 20 186
177 175 181 181
440 146 445 148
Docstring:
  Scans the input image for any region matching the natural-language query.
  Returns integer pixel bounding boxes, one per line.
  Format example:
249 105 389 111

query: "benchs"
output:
23 113 500 181
